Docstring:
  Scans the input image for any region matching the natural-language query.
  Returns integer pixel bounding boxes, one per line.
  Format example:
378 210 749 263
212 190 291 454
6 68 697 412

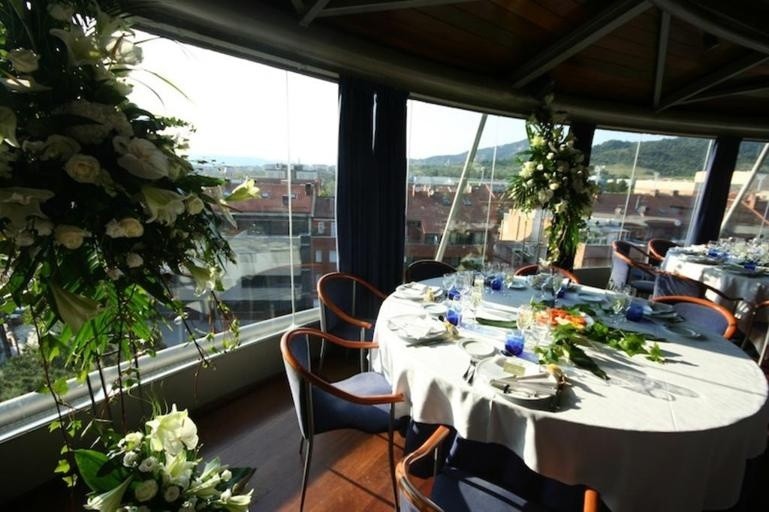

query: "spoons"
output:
463 356 477 380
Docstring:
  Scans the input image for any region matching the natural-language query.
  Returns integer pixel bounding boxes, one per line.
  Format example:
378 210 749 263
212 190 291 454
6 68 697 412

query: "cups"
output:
446 302 463 326
504 330 524 357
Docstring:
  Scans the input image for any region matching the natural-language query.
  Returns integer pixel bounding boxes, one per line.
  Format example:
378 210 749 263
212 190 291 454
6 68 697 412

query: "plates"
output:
577 291 603 303
640 301 678 319
423 303 447 314
458 337 496 357
475 355 558 401
660 322 703 339
396 283 444 300
387 313 447 341
713 265 766 275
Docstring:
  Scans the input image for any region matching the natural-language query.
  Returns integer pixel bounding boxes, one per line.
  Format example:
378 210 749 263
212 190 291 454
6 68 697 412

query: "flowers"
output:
476 292 669 382
0 1 261 512
501 98 601 250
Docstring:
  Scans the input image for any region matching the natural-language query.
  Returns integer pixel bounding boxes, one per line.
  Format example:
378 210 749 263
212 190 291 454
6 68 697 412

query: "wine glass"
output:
707 236 763 265
442 262 563 304
604 279 636 325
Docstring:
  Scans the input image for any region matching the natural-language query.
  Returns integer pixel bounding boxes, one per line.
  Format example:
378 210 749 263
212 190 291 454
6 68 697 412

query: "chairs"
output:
404 259 456 284
395 428 600 511
316 271 389 380
514 264 579 285
280 328 404 510
608 237 769 370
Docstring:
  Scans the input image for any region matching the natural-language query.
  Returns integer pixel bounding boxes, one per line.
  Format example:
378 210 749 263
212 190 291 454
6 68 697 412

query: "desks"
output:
367 271 768 511
